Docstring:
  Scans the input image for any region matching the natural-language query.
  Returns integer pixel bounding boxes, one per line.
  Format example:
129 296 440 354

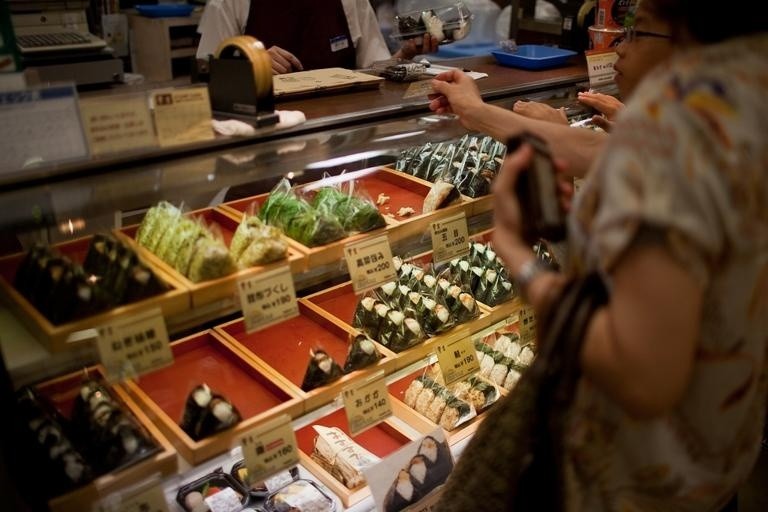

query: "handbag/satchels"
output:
430 266 607 511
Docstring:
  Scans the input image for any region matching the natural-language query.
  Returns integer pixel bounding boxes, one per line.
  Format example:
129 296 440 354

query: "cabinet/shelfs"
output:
2 70 588 511
125 14 204 81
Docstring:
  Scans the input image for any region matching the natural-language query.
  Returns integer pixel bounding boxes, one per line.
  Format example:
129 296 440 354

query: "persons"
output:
512 0 673 132
196 0 439 76
426 0 767 511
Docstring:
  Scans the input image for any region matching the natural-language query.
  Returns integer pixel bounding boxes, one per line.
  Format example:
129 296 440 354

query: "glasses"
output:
624 25 679 44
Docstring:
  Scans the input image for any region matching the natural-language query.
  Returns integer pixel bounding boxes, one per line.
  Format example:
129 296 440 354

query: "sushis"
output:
1 114 600 511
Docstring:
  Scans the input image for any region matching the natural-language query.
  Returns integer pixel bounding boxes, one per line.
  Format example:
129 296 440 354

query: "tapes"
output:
214 35 274 99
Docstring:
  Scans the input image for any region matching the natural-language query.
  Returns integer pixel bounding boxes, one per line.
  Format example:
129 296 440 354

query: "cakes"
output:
397 9 471 42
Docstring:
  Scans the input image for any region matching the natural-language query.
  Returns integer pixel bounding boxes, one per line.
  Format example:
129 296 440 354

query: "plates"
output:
135 4 197 19
488 45 579 72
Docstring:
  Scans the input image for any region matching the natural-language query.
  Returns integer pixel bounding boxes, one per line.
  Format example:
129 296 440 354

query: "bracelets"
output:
510 261 549 295
